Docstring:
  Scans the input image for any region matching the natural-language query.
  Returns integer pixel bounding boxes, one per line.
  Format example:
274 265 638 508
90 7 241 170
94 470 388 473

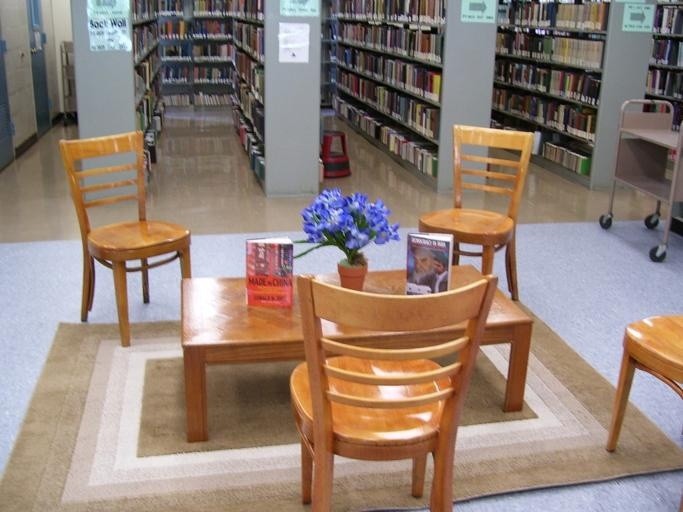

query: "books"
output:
246 237 293 308
406 232 454 295
490 1 682 174
322 1 446 178
132 2 263 184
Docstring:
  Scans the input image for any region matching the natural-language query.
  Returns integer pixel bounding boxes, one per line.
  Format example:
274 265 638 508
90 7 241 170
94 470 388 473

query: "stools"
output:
322 130 352 179
321 109 337 131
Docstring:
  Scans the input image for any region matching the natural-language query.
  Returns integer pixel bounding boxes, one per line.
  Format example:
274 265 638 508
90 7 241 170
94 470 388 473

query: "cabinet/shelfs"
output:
645 0 683 167
233 0 321 197
492 0 658 190
339 0 499 192
322 0 338 110
70 0 161 199
157 1 237 107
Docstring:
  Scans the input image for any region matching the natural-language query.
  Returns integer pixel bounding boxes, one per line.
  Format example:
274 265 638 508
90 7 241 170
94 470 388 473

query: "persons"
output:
409 247 448 293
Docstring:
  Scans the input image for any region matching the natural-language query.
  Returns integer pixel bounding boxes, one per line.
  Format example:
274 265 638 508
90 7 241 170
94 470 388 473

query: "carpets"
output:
1 299 683 511
1 218 682 510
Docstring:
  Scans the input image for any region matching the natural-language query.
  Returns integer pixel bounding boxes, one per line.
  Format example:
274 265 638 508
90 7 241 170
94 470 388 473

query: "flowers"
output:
292 188 401 264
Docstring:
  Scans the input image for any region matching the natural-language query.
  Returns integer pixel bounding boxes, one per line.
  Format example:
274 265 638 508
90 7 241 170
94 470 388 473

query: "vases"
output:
337 259 367 291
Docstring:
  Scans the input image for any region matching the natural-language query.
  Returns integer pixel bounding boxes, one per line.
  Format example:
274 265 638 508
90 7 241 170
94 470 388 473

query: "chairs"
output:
59 130 192 347
419 124 534 300
290 273 499 510
604 315 682 511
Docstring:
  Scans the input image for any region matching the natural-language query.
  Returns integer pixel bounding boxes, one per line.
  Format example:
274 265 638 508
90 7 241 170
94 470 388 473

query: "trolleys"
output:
598 98 682 263
